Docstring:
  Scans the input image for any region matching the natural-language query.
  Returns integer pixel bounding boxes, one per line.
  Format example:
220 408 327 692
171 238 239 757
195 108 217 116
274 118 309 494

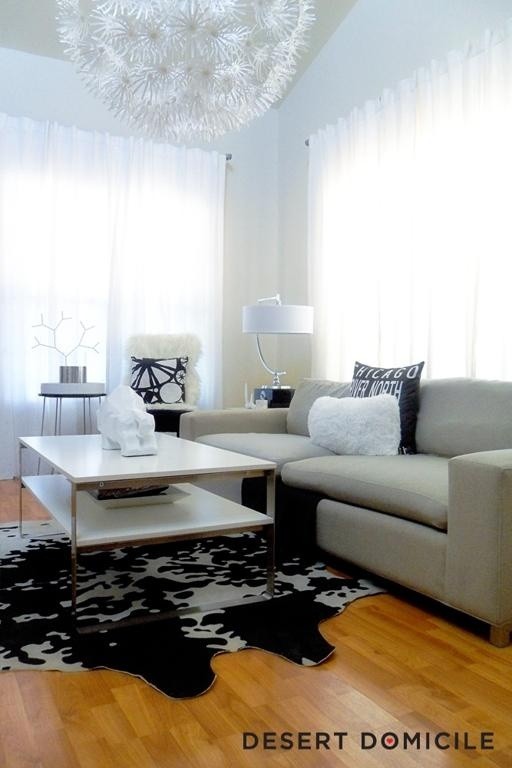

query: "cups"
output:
256 400 268 410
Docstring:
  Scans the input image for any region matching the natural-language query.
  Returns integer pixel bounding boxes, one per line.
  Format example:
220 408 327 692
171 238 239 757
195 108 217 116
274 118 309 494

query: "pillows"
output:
307 360 427 455
120 330 206 413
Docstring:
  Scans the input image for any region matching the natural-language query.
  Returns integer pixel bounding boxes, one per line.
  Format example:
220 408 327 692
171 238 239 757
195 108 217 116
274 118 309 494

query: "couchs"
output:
179 375 512 649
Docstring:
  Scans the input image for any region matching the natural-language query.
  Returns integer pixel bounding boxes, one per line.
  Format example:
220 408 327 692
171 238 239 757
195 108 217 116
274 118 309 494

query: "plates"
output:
82 479 191 510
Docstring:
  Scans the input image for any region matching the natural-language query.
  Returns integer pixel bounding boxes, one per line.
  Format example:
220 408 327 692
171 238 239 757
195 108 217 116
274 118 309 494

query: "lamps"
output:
241 291 315 409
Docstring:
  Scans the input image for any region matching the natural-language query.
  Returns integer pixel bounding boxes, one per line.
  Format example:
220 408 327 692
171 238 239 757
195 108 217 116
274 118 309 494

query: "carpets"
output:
1 514 390 704
